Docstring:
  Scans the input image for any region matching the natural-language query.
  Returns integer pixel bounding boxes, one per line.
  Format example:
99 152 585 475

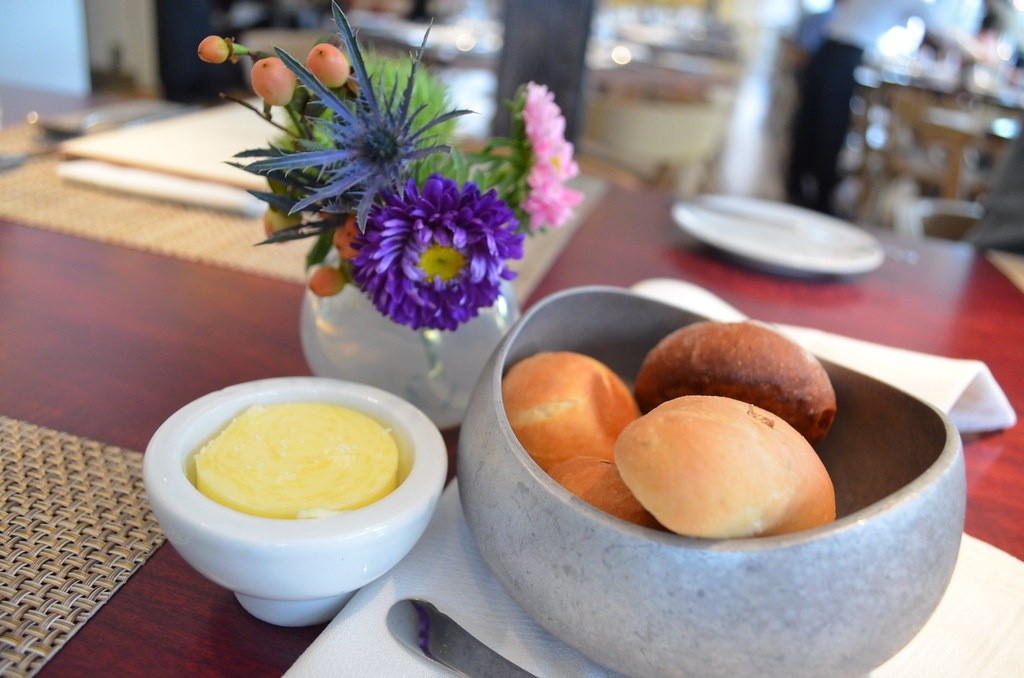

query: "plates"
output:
667 191 888 278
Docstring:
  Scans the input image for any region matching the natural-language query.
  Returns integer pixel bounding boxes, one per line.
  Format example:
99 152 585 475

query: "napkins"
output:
633 276 1017 431
64 163 272 216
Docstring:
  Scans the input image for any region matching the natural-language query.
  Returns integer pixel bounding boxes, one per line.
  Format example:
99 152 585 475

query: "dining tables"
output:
0 102 1024 678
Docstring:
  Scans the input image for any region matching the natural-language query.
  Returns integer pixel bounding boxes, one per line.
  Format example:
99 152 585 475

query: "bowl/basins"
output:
456 286 966 678
142 375 448 627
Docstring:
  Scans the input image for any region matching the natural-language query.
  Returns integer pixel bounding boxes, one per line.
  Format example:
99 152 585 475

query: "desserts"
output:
193 402 403 516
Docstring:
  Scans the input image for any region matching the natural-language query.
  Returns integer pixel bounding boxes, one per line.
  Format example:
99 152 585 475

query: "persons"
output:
759 0 1023 228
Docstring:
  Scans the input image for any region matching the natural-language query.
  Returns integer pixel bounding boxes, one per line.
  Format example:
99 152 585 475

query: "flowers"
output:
199 1 583 332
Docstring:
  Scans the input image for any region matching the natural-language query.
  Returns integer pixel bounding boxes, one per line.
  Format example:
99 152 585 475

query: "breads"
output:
502 321 839 539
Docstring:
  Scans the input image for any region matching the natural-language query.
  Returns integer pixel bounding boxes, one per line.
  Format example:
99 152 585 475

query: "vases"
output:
297 251 518 428
